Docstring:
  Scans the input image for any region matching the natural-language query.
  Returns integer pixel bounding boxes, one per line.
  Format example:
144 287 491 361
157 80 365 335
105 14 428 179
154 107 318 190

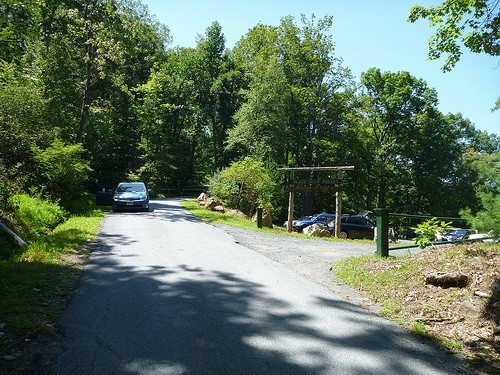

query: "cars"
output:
284 213 350 234
403 227 499 242
95 182 153 213
326 215 376 239
